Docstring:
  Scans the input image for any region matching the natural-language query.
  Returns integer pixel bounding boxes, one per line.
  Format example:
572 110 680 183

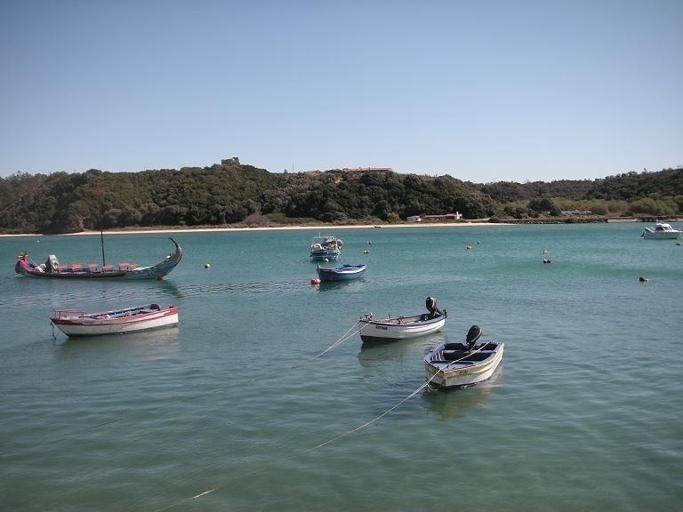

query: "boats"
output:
308 232 339 262
314 261 368 283
422 324 505 390
15 230 183 284
640 221 681 241
356 294 446 344
49 302 180 338
